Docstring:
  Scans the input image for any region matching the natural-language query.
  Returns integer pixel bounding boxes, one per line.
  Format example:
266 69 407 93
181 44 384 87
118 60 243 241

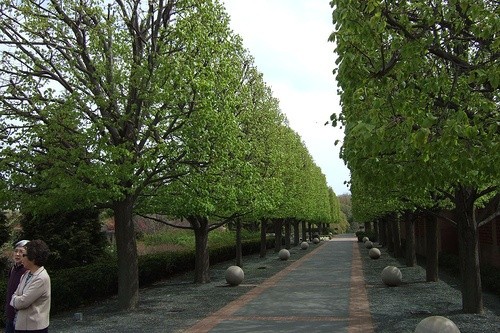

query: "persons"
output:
10 240 52 333
5 240 31 333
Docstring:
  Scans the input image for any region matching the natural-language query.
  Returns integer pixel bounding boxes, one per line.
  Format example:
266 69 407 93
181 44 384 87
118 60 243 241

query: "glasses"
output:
23 254 27 257
